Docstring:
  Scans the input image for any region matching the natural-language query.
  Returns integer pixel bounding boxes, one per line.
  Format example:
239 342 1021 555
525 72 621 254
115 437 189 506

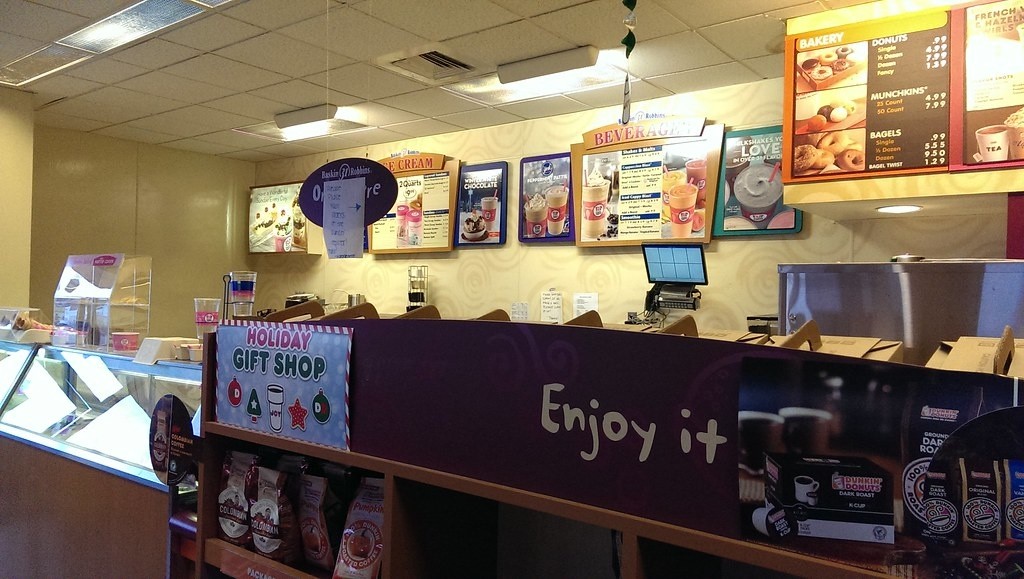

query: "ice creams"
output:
464 209 485 233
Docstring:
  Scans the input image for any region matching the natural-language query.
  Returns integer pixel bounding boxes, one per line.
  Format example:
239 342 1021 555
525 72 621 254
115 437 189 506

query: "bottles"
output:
348 294 360 308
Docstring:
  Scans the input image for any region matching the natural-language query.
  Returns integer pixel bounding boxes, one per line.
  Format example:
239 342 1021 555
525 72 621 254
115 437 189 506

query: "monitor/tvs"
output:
641 241 708 285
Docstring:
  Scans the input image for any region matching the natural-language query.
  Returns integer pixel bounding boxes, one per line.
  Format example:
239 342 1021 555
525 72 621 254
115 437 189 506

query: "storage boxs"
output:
600 305 1024 378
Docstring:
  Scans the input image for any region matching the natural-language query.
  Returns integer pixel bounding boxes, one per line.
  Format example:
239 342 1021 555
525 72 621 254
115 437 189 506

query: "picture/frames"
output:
711 123 803 238
518 152 575 243
455 159 508 246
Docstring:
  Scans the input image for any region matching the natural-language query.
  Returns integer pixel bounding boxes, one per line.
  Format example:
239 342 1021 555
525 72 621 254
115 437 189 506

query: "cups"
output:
397 205 409 238
276 230 292 252
481 197 498 231
406 209 422 245
805 492 818 505
669 183 699 237
583 179 611 237
974 120 1024 162
724 151 794 230
794 476 820 502
685 159 707 206
524 200 548 238
544 186 569 235
194 298 221 339
663 171 687 219
751 506 792 540
739 410 785 456
778 407 832 455
229 271 257 316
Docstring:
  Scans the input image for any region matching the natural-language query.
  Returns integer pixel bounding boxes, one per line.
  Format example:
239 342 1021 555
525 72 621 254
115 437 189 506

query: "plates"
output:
249 212 288 245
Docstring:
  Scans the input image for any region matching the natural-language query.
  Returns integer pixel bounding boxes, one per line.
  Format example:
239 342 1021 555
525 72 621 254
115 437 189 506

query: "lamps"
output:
496 47 601 84
274 104 339 128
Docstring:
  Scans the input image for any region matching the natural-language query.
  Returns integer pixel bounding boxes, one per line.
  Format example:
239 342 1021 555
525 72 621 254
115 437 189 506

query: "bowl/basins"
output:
189 349 203 361
463 227 487 239
110 331 140 350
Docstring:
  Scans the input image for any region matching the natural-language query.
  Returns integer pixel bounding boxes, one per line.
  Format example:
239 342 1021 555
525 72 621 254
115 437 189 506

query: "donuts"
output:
801 46 864 80
791 130 865 171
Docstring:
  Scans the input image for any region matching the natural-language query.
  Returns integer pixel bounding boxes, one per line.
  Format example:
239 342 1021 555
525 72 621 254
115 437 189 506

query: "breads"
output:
807 97 855 131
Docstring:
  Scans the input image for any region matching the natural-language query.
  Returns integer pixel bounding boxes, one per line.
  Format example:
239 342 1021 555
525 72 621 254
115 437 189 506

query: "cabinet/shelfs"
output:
195 332 896 579
52 255 150 353
0 334 202 579
